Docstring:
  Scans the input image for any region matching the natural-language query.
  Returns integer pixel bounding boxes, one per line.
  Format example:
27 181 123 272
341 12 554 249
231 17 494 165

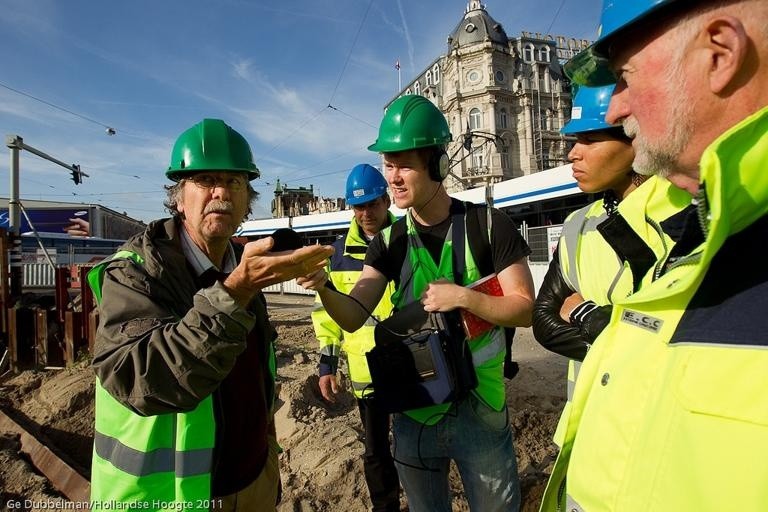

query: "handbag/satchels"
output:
366 296 479 415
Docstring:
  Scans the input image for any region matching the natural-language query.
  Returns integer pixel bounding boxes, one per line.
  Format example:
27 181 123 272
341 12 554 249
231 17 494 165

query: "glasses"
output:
178 173 248 191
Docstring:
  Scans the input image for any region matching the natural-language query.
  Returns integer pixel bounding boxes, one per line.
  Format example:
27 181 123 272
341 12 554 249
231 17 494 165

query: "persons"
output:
534 1 768 512
529 84 653 453
83 117 338 512
308 162 404 512
294 93 539 512
63 217 89 236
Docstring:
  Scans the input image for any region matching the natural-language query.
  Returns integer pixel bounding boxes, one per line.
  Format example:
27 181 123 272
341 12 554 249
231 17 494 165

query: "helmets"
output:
564 1 674 88
561 86 621 135
347 164 389 206
166 118 260 183
367 94 453 152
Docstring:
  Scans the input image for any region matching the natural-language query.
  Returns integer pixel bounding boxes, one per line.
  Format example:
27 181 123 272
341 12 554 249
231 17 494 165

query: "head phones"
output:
429 145 450 182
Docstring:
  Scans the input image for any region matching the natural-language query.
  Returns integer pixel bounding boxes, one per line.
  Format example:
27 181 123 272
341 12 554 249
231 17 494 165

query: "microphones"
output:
272 227 334 290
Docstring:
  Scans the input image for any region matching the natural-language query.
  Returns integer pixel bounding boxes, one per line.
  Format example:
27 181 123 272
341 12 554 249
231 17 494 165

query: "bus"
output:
19 229 128 264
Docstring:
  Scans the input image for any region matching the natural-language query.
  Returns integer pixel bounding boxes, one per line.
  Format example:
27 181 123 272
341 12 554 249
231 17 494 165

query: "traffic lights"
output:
69 163 79 185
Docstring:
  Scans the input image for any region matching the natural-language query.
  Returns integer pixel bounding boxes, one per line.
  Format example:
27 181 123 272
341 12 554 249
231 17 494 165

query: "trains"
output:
229 161 590 308
